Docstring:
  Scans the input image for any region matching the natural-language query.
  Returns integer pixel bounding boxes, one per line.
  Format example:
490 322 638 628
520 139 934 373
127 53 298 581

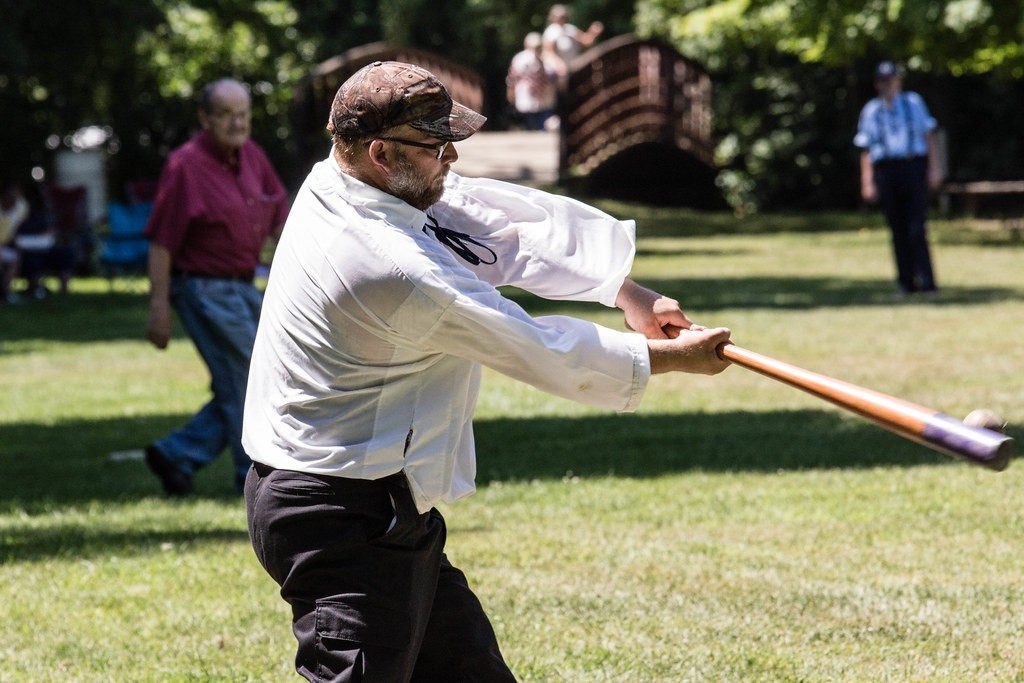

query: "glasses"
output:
362 136 450 159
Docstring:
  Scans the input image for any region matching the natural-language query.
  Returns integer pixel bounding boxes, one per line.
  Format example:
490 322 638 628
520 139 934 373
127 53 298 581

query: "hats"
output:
331 61 487 141
874 60 895 79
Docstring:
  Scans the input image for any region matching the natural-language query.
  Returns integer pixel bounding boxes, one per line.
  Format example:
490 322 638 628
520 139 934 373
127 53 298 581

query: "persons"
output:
542 5 603 75
143 79 292 498
506 32 558 130
853 61 945 293
0 171 40 301
241 60 732 683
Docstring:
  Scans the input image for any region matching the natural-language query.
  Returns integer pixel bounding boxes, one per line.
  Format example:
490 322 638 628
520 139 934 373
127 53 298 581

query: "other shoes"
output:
143 442 193 496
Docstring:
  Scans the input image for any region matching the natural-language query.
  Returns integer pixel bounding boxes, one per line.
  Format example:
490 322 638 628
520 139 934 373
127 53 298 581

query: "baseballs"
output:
963 408 1009 434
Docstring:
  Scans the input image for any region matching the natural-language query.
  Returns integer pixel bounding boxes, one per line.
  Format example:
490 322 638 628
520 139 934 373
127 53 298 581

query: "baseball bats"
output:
622 313 1016 474
